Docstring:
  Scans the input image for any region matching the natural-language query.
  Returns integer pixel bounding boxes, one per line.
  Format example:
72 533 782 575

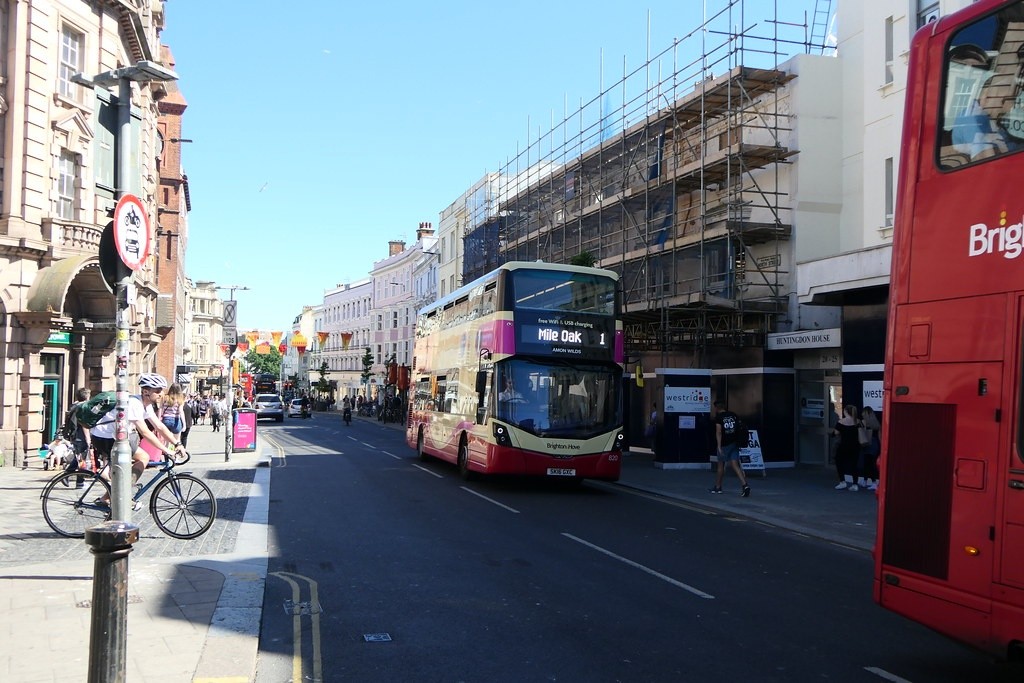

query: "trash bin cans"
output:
232 408 258 453
364 404 373 417
357 404 365 416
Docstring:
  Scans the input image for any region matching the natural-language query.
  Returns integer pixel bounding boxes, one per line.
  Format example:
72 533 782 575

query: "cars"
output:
286 398 313 418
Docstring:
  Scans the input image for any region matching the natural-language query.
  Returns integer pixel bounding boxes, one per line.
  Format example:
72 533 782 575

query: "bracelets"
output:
175 442 182 448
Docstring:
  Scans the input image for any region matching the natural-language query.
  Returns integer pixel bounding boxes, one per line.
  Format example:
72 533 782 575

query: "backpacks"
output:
77 389 139 430
62 404 80 441
729 411 749 448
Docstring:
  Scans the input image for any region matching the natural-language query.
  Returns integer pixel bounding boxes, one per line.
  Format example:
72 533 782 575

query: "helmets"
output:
138 373 168 389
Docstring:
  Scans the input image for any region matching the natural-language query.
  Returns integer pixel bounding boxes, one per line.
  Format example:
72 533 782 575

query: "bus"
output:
871 0 1024 648
408 260 645 486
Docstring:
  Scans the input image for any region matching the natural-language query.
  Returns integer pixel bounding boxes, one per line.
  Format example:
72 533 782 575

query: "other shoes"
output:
62 478 69 487
217 426 220 432
835 481 847 490
76 483 84 488
866 483 878 490
706 485 722 494
858 480 867 487
740 483 750 496
848 484 858 491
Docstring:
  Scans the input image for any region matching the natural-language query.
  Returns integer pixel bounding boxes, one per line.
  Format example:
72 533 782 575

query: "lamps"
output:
160 138 193 144
422 251 441 263
390 283 405 291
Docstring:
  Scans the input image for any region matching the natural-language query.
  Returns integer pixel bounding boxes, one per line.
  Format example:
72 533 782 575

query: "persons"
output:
498 375 530 404
302 392 335 411
706 400 750 497
45 434 68 471
158 382 187 458
61 387 91 489
188 390 240 432
650 401 657 452
342 395 379 423
89 372 186 511
836 405 881 492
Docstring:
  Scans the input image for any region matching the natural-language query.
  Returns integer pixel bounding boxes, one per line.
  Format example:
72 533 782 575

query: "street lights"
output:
215 285 252 463
84 59 175 682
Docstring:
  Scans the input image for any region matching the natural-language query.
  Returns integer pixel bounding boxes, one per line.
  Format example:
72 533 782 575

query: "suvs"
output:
252 393 285 423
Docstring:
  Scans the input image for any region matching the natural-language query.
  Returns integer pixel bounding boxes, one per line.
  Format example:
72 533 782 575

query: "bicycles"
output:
38 445 218 540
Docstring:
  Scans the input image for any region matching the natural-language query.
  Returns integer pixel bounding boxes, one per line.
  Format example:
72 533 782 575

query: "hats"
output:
55 435 61 440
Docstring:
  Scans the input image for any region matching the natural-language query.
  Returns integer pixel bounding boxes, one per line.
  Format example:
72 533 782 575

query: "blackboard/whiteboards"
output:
738 430 765 470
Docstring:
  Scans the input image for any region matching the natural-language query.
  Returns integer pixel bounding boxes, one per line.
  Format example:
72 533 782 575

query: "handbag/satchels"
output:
74 446 97 477
858 420 873 445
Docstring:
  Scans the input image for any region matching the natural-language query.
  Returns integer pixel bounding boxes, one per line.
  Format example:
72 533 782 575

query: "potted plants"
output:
357 347 376 416
315 361 333 412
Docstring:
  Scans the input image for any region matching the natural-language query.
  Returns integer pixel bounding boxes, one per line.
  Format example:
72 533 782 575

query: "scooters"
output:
343 408 352 427
